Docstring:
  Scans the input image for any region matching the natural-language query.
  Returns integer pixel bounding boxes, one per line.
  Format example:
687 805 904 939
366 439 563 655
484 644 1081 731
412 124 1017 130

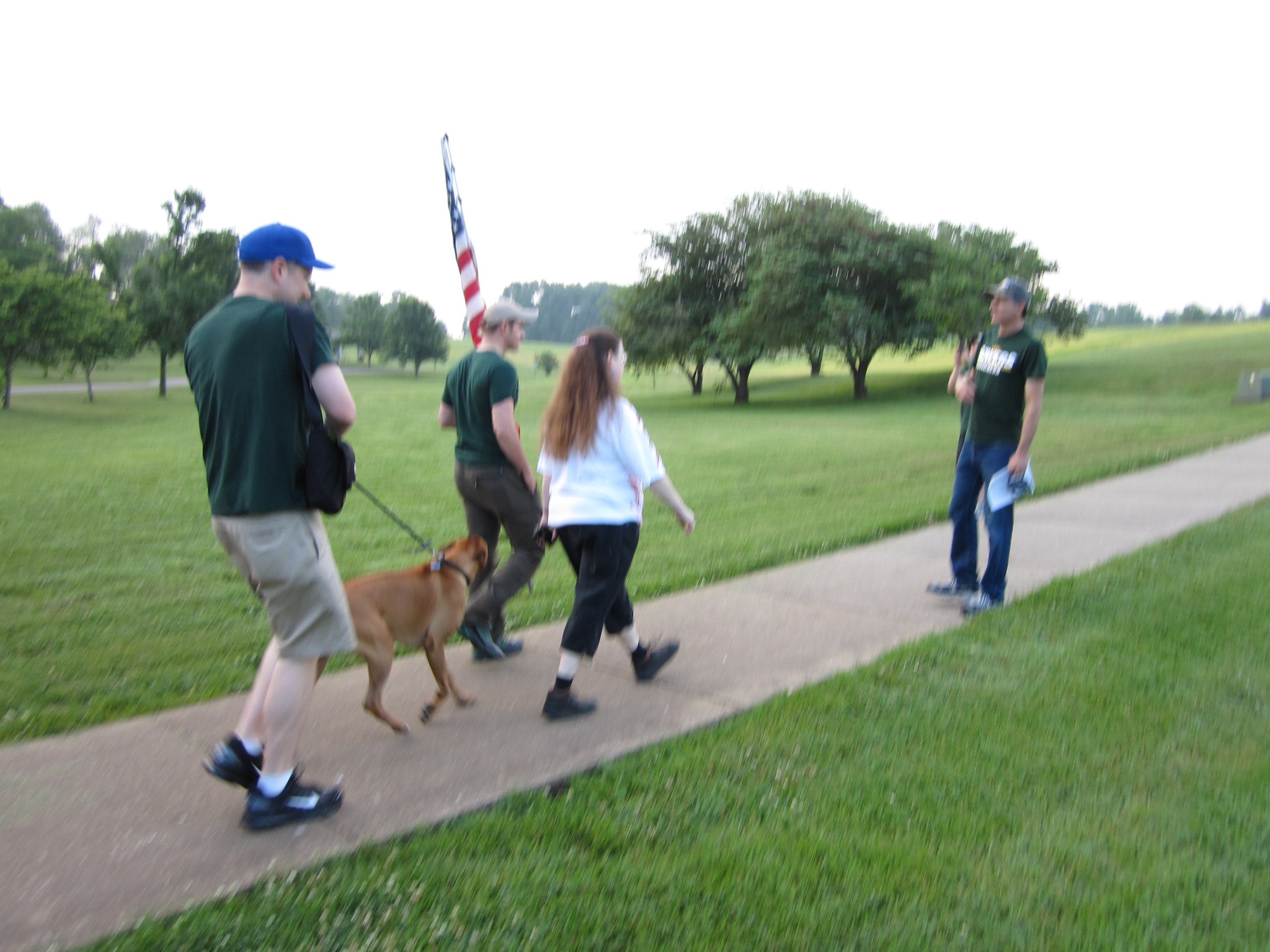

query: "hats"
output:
236 222 333 270
480 298 540 325
984 278 1029 308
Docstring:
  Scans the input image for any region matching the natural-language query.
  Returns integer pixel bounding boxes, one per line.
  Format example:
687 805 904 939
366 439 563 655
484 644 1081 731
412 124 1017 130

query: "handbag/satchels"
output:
283 300 359 515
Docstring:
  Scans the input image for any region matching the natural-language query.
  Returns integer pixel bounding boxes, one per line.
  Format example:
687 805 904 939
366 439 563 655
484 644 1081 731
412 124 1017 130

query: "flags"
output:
440 135 487 350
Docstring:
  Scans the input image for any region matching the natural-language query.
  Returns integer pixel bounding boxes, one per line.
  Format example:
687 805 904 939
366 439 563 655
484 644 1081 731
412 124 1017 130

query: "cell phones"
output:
537 526 553 545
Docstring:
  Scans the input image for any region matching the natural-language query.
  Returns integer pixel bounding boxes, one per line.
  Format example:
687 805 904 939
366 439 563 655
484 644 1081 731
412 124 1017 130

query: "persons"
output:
923 278 1048 615
439 301 546 663
539 328 696 721
184 222 356 833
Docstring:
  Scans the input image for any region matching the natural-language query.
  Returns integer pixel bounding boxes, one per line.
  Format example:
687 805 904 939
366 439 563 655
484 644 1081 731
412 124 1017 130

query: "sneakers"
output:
202 737 263 791
244 763 342 832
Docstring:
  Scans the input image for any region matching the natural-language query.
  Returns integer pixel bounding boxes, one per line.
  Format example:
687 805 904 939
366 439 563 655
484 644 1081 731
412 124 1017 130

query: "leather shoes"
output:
474 636 524 661
459 617 506 662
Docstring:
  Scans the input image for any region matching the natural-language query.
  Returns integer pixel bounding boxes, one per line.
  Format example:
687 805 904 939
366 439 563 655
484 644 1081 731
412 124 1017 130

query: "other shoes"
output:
541 695 594 718
959 593 1005 616
925 578 975 596
637 634 680 684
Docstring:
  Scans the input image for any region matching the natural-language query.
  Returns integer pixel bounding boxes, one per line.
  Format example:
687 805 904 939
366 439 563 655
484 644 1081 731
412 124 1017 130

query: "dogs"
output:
307 534 489 733
